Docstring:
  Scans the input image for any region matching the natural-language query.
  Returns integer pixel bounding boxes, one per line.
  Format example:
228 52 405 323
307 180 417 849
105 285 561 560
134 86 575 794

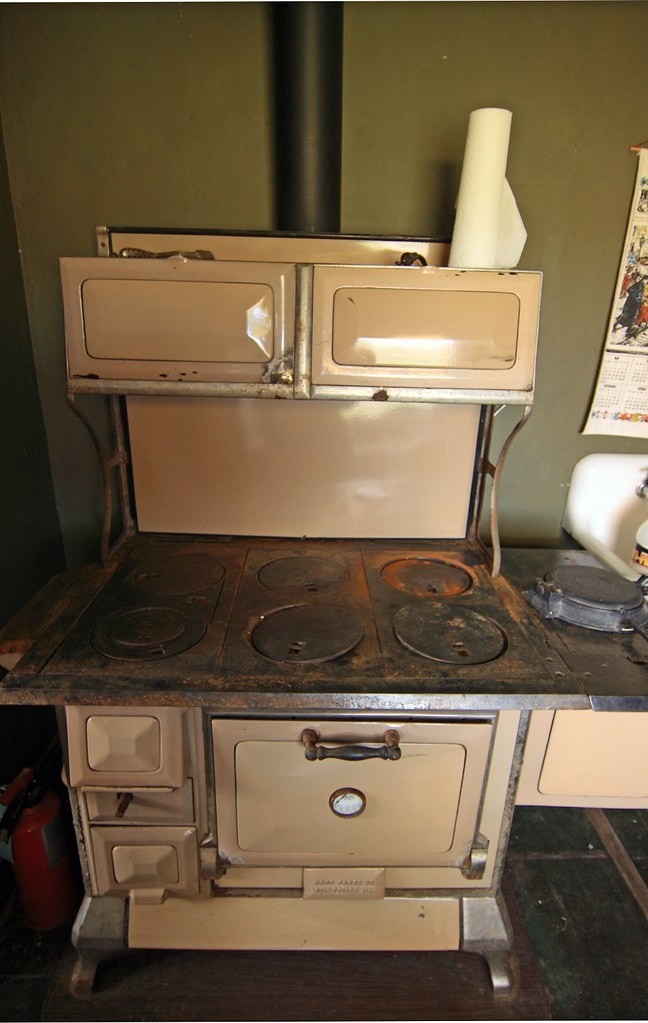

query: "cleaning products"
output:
0 767 78 932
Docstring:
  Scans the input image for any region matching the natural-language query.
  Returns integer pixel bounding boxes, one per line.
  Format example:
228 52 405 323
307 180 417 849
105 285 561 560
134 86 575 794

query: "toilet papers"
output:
448 107 528 269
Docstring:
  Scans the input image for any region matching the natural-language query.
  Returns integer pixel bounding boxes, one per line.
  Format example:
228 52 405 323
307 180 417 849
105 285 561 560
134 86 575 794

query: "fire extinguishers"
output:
0 767 77 933
